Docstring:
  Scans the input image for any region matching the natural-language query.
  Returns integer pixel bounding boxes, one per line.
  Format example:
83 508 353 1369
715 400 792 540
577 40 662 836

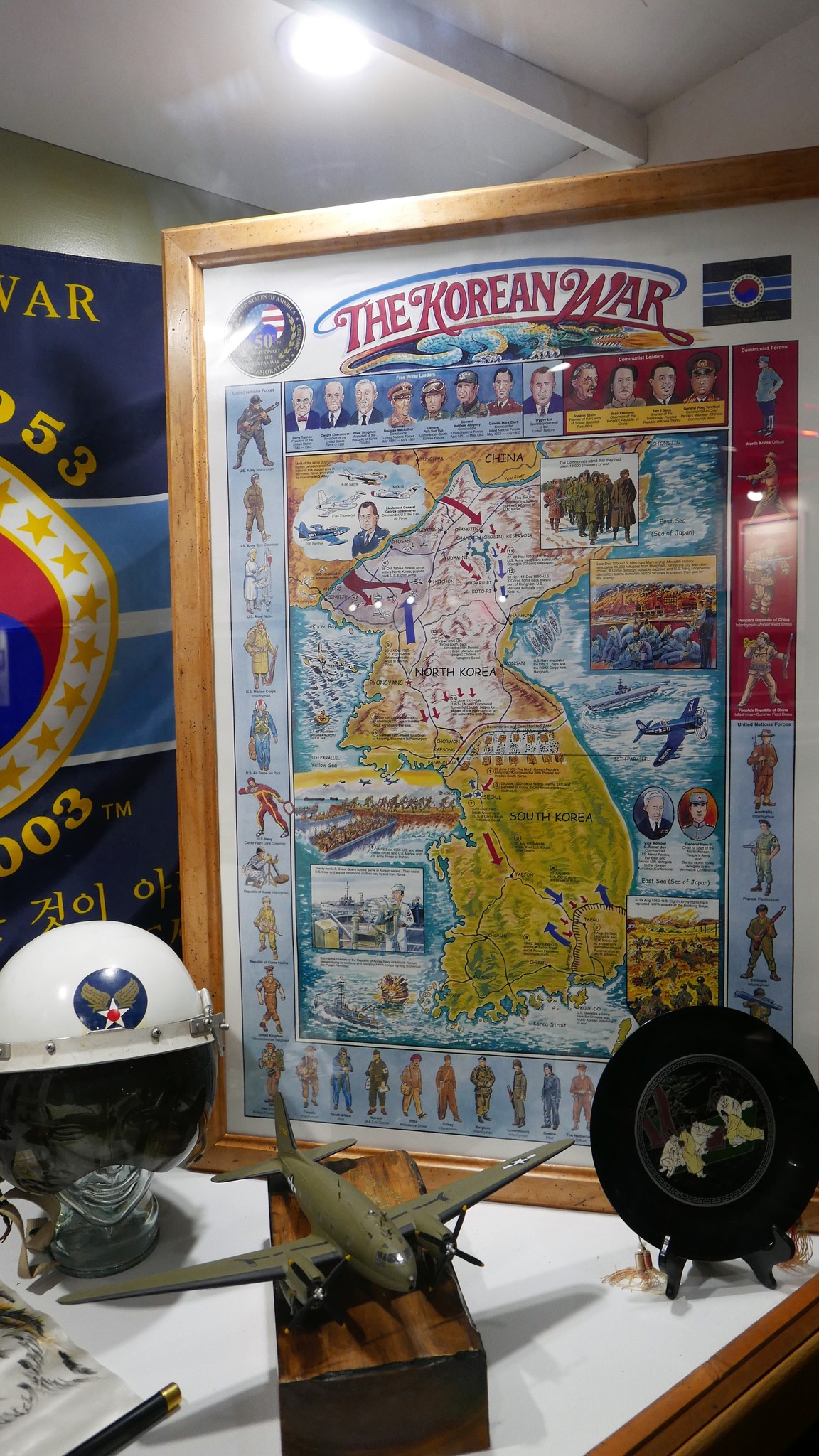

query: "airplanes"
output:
54 1088 576 1337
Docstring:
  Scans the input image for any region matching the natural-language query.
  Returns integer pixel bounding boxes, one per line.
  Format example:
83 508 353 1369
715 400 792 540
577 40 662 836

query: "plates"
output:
589 1005 819 1262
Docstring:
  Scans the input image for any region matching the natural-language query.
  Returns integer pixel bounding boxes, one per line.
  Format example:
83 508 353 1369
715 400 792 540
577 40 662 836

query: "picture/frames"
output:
159 143 819 1236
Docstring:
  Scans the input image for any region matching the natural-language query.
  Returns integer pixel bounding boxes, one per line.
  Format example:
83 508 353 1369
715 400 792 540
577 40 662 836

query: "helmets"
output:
0 920 230 1195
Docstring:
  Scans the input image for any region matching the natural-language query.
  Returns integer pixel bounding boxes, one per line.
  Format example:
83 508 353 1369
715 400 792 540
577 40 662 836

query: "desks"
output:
0 1168 819 1456
